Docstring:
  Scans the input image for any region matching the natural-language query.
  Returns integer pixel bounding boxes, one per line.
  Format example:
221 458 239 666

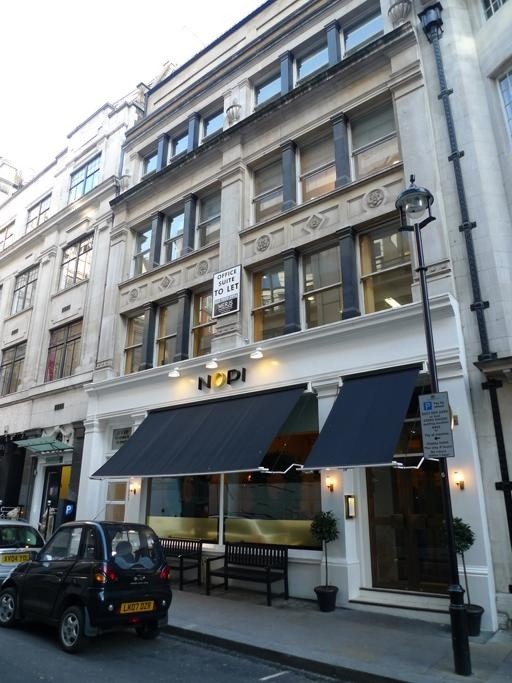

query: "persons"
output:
113 540 134 560
2 528 31 546
37 499 55 541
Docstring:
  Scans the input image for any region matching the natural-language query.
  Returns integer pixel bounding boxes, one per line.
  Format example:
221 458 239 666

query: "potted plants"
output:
309 512 341 612
440 516 486 639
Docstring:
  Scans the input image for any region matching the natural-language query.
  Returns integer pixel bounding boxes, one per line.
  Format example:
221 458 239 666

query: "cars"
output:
0 519 52 583
0 521 172 654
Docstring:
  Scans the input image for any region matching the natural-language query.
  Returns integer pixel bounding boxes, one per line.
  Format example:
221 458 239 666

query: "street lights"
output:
395 175 471 676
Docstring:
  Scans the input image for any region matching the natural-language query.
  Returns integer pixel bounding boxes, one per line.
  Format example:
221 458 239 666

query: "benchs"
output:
157 537 290 606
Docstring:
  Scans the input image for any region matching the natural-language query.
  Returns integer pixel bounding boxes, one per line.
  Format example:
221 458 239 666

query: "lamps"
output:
168 346 263 379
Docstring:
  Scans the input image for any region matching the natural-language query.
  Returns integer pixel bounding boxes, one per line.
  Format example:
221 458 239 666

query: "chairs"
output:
113 541 135 563
